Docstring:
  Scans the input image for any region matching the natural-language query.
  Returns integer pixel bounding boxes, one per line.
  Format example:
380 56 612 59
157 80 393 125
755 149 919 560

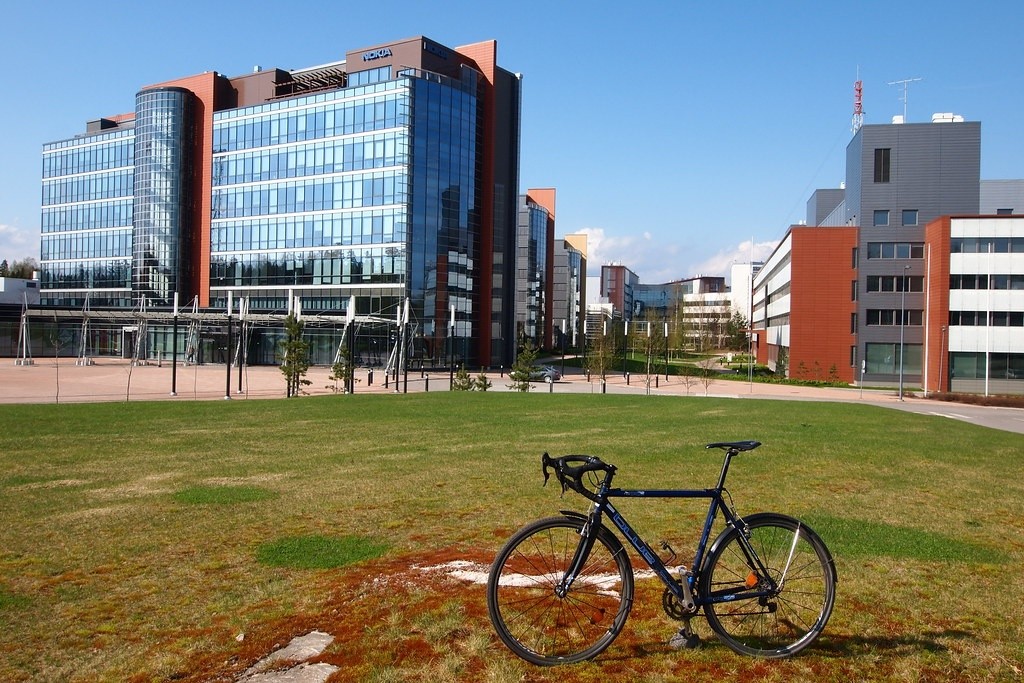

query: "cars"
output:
508 363 561 383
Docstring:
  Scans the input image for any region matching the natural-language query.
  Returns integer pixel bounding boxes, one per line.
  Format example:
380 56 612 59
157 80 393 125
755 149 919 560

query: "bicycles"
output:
485 440 837 666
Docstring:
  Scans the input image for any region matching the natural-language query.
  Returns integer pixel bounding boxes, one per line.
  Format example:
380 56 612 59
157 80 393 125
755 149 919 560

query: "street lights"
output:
899 265 912 400
